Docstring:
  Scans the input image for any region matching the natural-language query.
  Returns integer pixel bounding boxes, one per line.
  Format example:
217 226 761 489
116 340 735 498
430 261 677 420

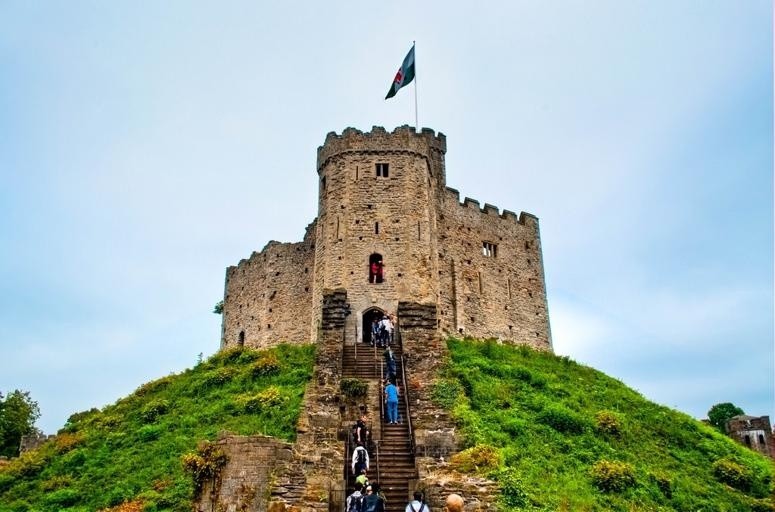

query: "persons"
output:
443 492 466 512
370 309 401 425
370 260 380 284
345 413 389 511
404 490 430 512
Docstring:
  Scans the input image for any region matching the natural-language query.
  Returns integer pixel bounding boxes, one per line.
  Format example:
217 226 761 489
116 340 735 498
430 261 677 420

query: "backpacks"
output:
348 494 363 512
355 449 366 464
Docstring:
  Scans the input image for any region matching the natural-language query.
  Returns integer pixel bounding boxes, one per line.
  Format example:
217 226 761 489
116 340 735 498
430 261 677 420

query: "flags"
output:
384 38 417 101
